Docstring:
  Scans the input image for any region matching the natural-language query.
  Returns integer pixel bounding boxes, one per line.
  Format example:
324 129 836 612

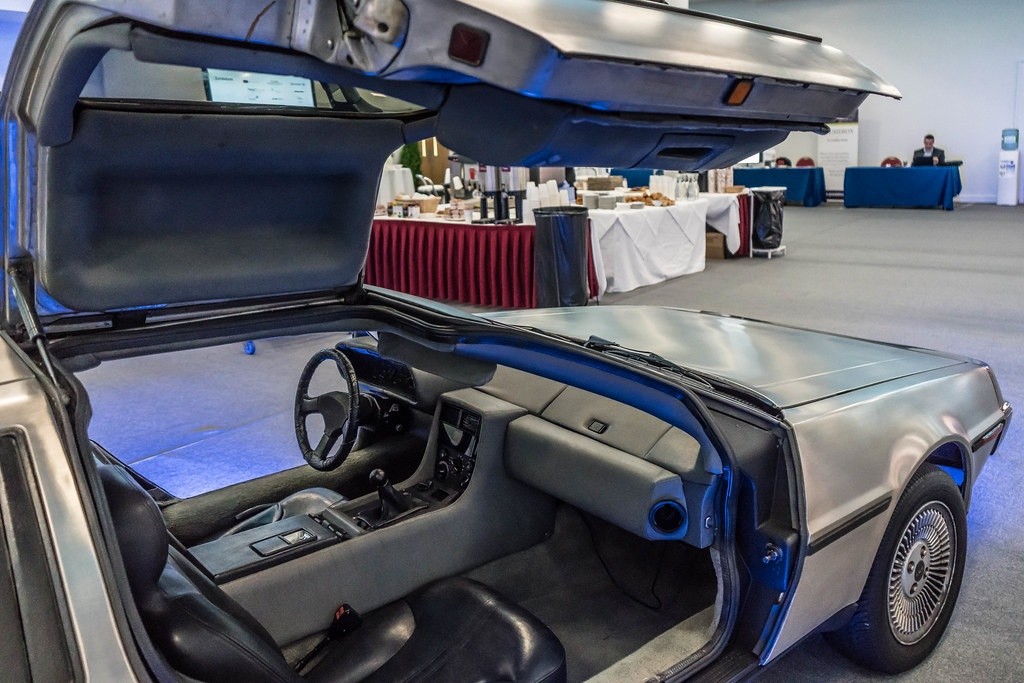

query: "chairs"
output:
880 156 904 167
776 156 792 167
795 156 814 167
90 462 572 683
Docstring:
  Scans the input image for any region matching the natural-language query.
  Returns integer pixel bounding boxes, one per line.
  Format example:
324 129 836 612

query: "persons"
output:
912 135 944 166
777 158 785 165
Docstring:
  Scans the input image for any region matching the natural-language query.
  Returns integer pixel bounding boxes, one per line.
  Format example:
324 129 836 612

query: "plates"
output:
579 190 663 211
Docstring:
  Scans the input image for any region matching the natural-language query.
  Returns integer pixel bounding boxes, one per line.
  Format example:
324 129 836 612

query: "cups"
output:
453 176 464 191
524 179 569 216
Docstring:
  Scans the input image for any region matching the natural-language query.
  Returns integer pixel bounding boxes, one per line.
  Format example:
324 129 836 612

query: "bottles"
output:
675 174 701 200
386 201 463 219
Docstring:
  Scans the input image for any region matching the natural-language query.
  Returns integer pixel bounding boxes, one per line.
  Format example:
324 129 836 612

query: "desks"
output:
842 164 963 211
733 165 828 206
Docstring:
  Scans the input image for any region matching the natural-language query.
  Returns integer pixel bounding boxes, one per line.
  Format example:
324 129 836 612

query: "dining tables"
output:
364 185 706 307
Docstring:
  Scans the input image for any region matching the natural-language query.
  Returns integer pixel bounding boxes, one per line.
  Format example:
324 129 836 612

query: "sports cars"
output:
0 0 1009 682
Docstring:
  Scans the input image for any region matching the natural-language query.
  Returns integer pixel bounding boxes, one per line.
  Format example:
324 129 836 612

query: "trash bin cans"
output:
751 186 788 260
534 205 589 308
